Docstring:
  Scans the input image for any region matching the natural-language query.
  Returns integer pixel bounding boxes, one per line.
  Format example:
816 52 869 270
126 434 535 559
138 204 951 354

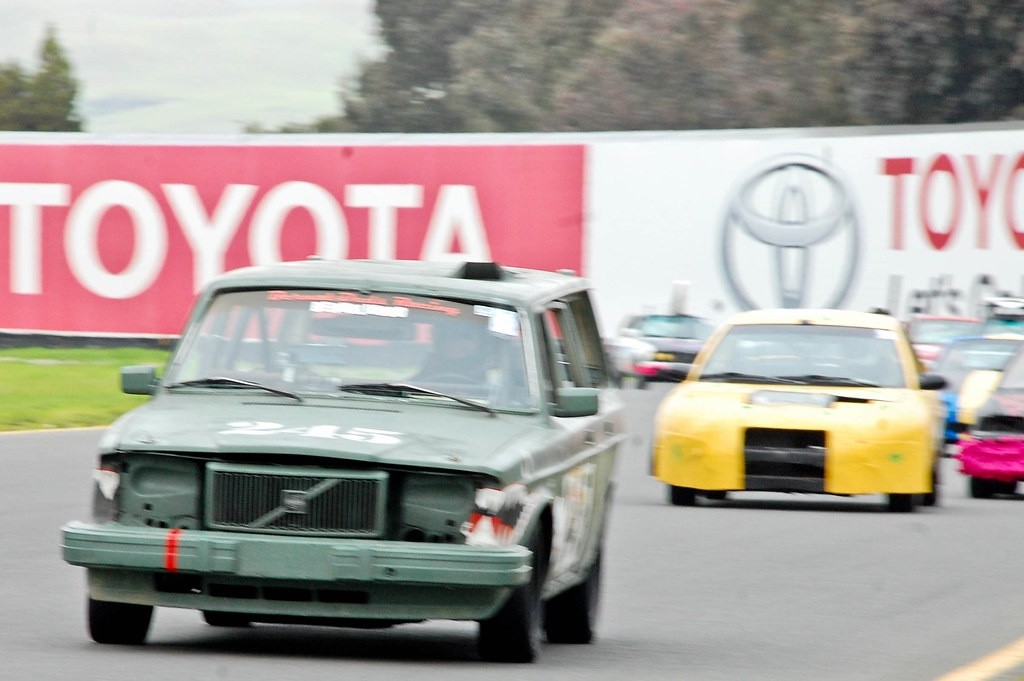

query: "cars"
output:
904 294 1024 498
61 258 626 665
606 311 715 387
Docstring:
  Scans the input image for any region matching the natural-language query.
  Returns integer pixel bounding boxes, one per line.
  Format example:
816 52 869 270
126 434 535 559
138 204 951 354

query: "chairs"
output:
291 341 352 366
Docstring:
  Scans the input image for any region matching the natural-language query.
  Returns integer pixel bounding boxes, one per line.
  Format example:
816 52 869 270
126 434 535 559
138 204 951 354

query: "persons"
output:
409 316 520 396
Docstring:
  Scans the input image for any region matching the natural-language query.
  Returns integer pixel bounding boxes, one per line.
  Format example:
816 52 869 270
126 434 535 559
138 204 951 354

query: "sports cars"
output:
651 306 948 511
